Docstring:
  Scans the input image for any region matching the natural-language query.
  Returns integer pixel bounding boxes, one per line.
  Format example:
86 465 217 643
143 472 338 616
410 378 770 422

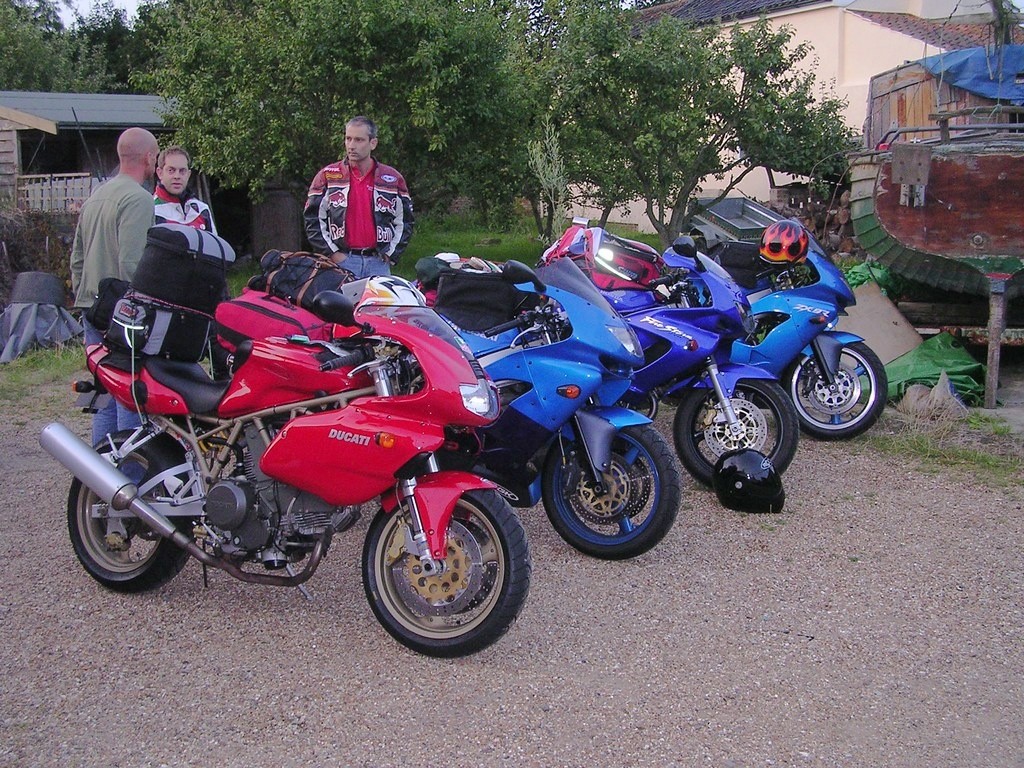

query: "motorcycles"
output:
395 254 677 559
37 266 533 660
556 234 802 488
592 212 885 443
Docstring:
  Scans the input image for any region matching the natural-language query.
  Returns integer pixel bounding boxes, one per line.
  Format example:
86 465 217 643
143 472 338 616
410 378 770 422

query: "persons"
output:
303 115 414 279
69 127 158 484
153 148 218 236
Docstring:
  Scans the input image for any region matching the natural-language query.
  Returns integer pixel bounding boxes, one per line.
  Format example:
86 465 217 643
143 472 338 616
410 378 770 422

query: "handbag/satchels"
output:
246 248 359 312
86 222 238 363
433 266 516 332
589 235 668 291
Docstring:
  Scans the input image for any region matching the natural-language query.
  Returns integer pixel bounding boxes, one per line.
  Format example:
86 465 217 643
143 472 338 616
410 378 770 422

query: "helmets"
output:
714 448 785 514
758 220 808 267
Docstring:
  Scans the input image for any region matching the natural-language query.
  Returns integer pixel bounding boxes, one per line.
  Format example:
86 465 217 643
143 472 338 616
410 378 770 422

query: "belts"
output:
342 248 380 256
